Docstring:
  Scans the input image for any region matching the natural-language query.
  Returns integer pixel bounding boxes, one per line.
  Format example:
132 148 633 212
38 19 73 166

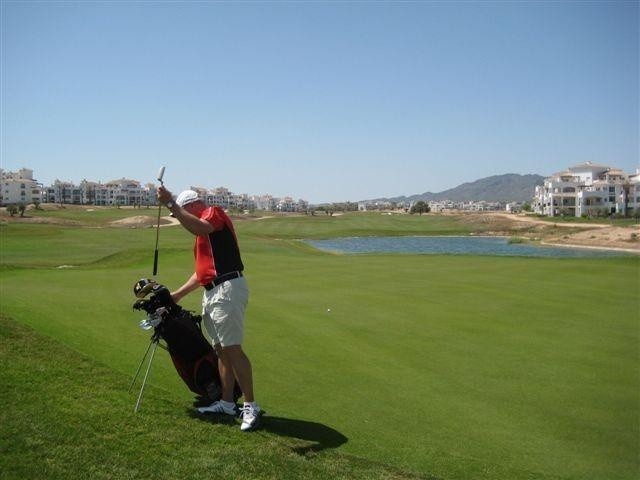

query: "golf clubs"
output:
154 167 166 275
132 278 176 332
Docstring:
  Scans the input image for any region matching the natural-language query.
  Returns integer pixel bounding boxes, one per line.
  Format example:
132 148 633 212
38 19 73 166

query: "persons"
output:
157 186 262 431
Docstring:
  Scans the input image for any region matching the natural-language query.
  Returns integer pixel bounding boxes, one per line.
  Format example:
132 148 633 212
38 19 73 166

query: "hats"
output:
169 189 203 217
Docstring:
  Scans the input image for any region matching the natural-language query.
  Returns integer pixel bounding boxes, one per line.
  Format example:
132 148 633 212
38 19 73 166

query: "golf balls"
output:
327 309 331 312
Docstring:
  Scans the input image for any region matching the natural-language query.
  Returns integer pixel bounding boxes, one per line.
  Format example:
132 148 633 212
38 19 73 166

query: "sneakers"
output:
240 405 261 431
198 401 238 415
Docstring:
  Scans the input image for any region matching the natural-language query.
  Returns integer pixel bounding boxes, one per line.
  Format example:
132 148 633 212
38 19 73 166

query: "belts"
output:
204 271 244 291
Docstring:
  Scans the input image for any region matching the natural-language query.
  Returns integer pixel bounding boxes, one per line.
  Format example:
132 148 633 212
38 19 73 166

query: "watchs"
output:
166 200 175 209
